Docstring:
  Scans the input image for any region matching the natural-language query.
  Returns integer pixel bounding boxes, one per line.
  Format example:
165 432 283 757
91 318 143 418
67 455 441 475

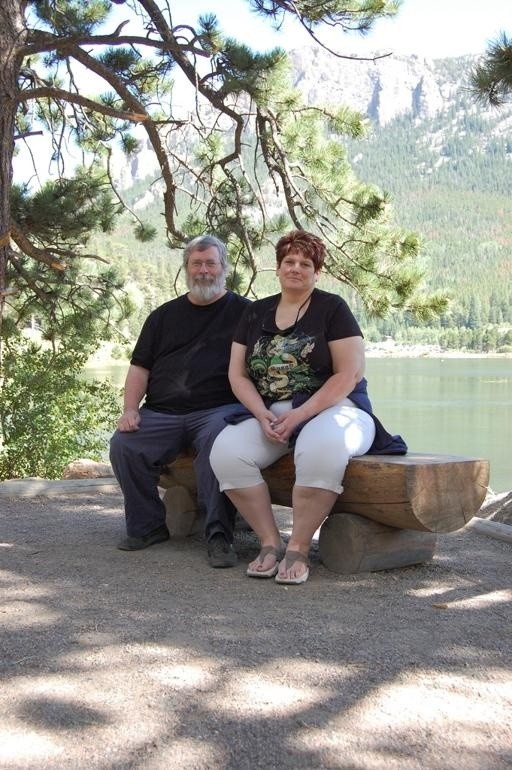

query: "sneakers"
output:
119 523 169 550
208 535 238 567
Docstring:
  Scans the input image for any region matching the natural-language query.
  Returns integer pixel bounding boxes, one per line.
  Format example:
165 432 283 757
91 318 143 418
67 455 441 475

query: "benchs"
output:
153 445 492 574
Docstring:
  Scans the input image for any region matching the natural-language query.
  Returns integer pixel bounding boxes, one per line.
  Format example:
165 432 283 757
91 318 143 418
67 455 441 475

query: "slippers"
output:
275 551 310 584
247 546 285 577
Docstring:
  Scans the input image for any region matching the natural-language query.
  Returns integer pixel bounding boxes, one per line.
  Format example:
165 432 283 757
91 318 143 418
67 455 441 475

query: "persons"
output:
209 230 407 585
110 235 255 568
